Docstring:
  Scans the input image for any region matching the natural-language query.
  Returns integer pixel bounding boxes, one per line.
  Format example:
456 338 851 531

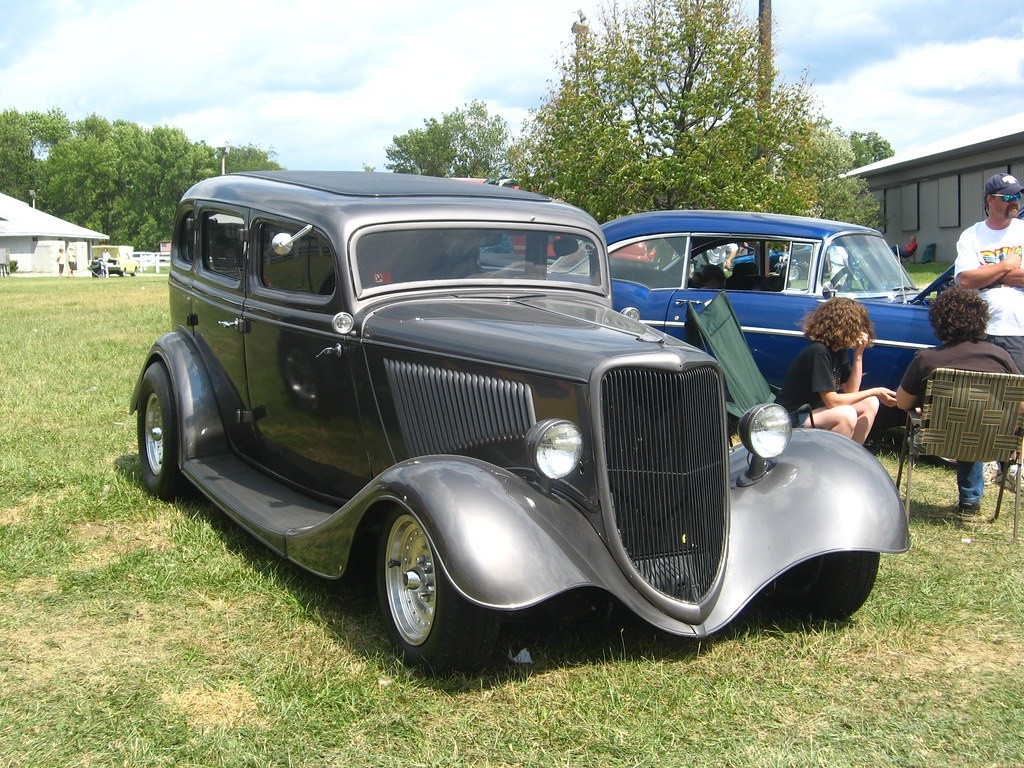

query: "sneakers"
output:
1006 464 1024 492
957 504 980 517
982 461 1003 485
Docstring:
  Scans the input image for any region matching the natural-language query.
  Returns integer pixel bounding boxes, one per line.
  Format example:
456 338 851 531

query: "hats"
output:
986 173 1022 196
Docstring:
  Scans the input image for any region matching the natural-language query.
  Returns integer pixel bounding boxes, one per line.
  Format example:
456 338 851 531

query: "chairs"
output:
688 263 791 293
896 368 1024 545
900 246 918 264
89 261 104 279
684 290 815 447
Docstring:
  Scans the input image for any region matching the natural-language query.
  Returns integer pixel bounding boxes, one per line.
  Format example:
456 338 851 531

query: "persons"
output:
892 235 918 258
895 285 1022 522
56 246 112 278
689 242 747 274
953 173 1024 496
772 294 897 446
826 241 850 290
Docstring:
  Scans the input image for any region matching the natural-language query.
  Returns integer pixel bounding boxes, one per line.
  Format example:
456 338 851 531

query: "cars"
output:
446 177 1023 439
88 244 138 277
127 169 913 672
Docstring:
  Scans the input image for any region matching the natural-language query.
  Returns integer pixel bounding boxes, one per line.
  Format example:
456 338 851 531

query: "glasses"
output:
992 193 1023 202
